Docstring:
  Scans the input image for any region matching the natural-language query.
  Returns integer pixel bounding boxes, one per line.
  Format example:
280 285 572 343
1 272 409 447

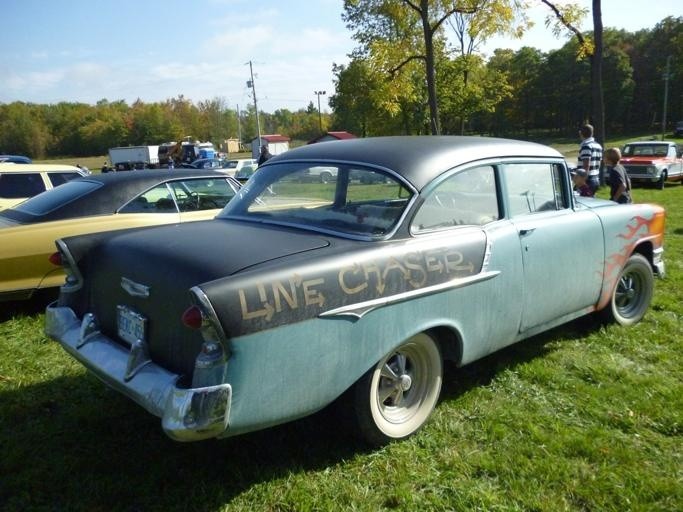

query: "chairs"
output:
356 203 489 235
137 197 175 213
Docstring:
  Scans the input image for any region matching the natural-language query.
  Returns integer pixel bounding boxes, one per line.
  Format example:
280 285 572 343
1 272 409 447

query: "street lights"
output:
244 60 262 156
313 90 325 130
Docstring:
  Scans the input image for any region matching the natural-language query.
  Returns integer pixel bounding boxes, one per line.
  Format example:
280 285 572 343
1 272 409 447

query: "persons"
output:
570 168 592 197
76 163 92 175
573 123 604 199
100 162 108 173
128 163 136 172
167 155 174 170
257 144 276 197
599 146 633 205
